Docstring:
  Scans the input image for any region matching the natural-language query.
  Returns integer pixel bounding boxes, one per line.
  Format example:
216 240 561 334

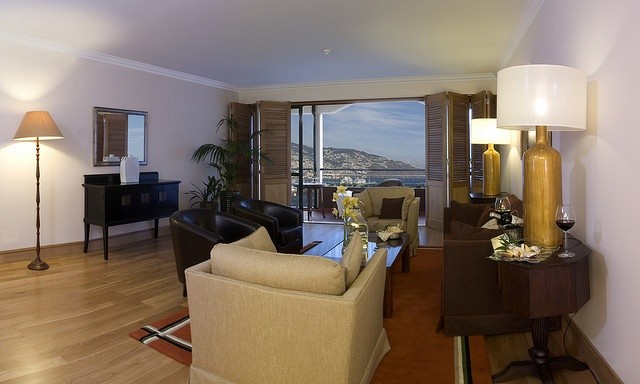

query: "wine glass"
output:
494 197 512 216
554 205 576 258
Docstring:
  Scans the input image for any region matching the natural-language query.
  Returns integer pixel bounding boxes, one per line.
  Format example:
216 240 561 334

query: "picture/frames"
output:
520 129 552 159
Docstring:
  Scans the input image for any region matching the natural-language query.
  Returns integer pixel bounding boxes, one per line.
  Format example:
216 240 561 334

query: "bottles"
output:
489 212 523 225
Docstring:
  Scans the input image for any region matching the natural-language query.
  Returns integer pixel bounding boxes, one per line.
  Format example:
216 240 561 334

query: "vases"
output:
342 216 348 253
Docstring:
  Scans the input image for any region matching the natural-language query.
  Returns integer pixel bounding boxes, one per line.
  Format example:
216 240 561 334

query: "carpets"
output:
129 241 492 384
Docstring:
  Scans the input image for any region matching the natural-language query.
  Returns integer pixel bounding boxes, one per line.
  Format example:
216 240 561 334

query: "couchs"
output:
230 197 303 253
353 186 420 257
169 207 262 297
435 192 564 336
185 226 392 383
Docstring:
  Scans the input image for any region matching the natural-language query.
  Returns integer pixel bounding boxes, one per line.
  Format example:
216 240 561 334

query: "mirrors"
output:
92 105 148 166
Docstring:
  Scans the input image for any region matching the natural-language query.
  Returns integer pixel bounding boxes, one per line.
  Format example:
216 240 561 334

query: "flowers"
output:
332 185 361 219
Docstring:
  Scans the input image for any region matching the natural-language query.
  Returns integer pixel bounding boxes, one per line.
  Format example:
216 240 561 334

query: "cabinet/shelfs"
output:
493 235 592 381
82 171 181 258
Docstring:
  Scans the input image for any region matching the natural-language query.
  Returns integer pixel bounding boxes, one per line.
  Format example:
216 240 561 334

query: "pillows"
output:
209 243 345 294
448 199 480 225
379 197 405 218
340 229 364 288
229 226 278 252
449 219 499 241
477 205 495 228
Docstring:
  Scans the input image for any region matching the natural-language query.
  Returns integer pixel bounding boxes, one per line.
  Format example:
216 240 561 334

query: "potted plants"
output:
182 176 225 210
191 114 276 210
208 181 222 211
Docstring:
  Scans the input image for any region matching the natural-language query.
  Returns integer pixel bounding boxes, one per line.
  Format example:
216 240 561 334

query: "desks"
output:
293 183 327 221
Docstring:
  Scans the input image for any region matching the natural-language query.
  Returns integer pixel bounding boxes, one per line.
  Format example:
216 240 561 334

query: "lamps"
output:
14 109 65 270
469 117 510 196
496 63 588 247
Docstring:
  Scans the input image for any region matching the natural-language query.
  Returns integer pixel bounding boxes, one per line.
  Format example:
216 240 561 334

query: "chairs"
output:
378 177 404 187
